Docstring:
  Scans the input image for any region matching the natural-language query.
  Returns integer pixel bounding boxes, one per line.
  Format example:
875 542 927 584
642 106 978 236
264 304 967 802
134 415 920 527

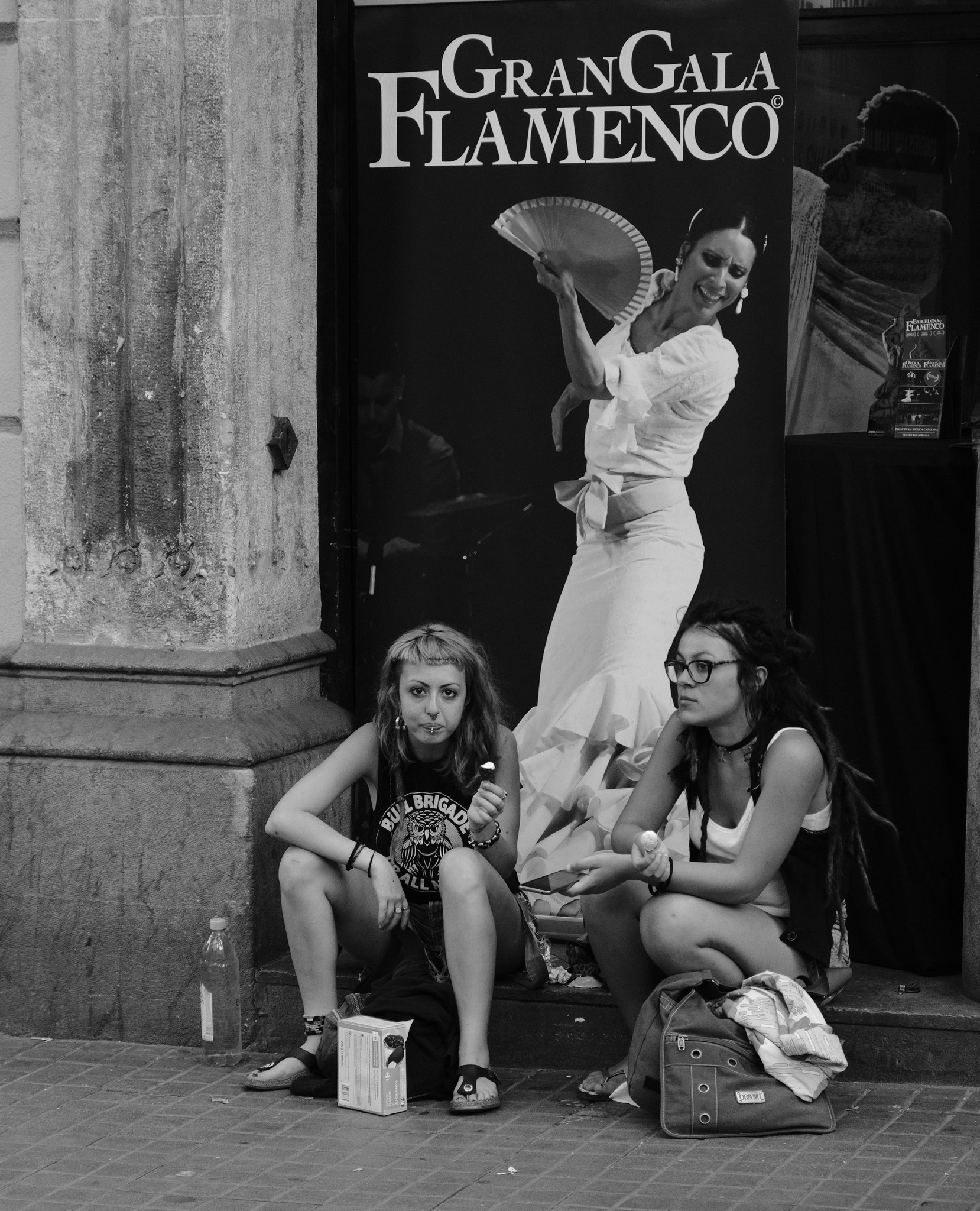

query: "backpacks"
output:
315 992 373 1083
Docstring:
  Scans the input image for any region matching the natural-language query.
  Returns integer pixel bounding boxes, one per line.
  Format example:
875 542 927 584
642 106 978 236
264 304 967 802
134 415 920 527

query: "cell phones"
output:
520 869 586 894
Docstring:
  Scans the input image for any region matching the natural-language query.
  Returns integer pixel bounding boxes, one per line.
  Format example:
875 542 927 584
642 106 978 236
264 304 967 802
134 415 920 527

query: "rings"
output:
395 907 402 913
501 790 508 800
403 905 409 911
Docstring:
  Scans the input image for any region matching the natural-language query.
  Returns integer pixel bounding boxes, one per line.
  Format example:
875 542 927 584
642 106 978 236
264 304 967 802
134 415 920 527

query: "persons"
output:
510 199 769 918
244 625 534 1112
559 597 894 1094
785 85 960 433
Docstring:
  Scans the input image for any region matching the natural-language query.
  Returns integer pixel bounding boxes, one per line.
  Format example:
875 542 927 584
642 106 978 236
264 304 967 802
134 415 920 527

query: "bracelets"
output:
469 824 487 833
467 820 501 848
368 851 376 877
345 840 365 870
648 857 673 895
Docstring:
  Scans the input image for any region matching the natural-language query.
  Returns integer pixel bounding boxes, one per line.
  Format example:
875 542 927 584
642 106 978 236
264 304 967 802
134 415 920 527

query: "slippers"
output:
449 1065 501 1112
576 1068 626 1101
244 1046 319 1090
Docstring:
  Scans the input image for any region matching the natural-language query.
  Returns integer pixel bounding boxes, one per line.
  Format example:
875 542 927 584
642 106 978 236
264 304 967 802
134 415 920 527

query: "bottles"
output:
199 918 243 1067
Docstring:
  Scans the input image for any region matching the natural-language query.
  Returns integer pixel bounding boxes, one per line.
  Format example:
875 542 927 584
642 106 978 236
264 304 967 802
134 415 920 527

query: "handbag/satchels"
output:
627 970 836 1139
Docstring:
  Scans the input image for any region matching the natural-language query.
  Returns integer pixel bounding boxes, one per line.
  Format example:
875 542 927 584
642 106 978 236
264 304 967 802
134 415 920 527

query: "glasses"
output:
663 659 741 683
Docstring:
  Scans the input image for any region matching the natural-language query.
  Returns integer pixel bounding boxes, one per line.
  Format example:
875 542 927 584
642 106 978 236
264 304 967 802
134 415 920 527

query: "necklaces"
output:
709 730 756 763
